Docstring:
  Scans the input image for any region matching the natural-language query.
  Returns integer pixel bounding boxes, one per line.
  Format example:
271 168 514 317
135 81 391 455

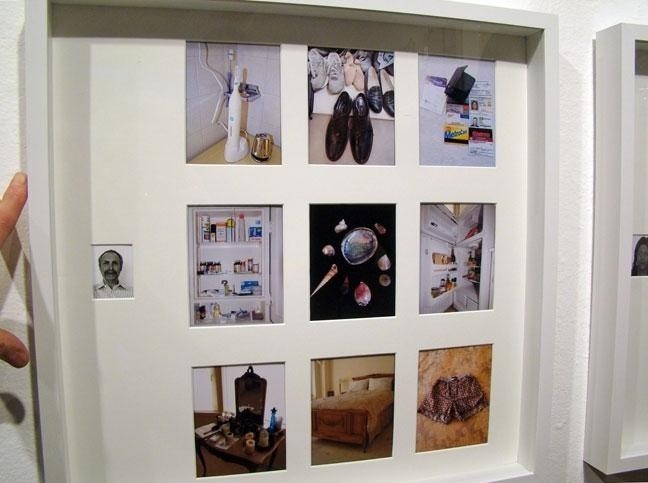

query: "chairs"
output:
234 365 268 427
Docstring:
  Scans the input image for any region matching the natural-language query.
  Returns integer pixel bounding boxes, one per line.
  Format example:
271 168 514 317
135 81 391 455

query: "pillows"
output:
347 377 393 393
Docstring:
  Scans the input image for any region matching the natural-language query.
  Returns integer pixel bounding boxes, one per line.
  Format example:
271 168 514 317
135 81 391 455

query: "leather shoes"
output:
308 48 394 164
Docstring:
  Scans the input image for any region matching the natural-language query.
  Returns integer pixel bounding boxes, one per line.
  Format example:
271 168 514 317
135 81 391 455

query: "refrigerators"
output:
419 203 495 314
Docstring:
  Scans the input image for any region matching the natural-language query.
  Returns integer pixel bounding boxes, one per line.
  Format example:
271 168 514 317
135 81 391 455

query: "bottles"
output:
210 303 220 318
234 257 259 273
259 429 270 447
450 248 455 263
269 408 277 432
219 284 232 295
439 274 456 294
201 215 227 242
238 213 246 241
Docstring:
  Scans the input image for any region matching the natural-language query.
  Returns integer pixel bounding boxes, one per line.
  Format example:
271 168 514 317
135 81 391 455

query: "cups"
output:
245 440 255 454
221 423 233 445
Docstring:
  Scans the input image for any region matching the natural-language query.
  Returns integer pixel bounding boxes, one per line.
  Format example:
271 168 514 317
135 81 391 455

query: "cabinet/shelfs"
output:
191 207 272 325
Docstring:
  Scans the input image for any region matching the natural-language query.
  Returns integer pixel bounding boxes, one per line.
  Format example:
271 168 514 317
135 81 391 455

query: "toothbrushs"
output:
225 50 233 105
223 64 250 163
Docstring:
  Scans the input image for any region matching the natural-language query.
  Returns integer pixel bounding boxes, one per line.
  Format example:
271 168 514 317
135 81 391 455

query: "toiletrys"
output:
247 439 256 453
269 408 278 430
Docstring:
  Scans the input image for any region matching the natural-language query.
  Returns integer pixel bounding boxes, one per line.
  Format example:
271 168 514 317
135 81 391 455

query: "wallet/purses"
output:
447 66 476 101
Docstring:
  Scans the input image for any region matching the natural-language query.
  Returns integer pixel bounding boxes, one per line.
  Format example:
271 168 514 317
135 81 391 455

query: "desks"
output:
194 420 286 473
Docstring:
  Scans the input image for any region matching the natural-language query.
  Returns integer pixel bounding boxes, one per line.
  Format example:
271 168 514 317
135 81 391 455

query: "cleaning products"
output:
237 214 247 241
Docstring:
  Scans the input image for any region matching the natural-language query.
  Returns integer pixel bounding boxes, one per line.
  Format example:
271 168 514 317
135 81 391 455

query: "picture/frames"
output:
24 0 558 483
581 22 648 477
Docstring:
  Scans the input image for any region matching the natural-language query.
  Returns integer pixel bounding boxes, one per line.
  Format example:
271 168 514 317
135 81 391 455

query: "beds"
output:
311 373 395 453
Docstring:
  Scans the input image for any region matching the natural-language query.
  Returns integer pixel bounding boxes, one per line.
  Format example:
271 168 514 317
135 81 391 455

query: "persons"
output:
470 116 479 127
0 172 31 368
92 249 132 298
470 101 479 112
462 104 468 115
